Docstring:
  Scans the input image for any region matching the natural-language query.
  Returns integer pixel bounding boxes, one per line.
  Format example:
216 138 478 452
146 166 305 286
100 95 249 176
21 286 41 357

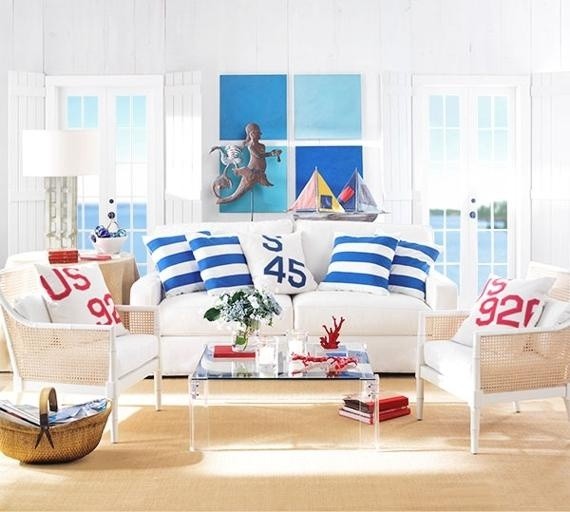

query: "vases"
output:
231 326 249 353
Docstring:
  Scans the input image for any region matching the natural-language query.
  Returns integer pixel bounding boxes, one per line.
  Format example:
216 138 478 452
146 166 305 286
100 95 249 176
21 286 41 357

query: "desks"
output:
7 252 140 331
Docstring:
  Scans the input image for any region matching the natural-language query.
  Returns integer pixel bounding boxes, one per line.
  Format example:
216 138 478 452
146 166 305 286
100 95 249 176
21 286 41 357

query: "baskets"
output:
0 388 112 464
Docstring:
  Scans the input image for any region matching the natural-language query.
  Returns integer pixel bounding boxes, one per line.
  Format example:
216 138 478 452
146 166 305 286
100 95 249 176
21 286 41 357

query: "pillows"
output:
388 237 444 302
141 230 210 299
318 228 404 297
237 232 318 296
450 273 556 350
184 229 255 296
33 261 129 348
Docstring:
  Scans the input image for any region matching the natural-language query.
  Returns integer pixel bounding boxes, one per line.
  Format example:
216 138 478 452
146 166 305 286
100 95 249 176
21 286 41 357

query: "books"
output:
337 407 411 425
342 405 409 418
343 392 409 413
78 254 112 262
47 249 80 264
0 399 107 428
213 345 256 359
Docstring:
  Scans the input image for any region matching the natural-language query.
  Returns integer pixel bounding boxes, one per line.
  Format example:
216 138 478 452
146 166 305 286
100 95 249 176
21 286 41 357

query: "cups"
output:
256 338 278 372
287 327 310 358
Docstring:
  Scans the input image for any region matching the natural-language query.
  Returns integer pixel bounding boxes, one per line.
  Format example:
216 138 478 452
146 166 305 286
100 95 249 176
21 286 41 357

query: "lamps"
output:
23 129 106 248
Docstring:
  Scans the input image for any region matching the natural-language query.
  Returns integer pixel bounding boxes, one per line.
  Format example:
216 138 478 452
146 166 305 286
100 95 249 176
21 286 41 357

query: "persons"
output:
211 123 282 204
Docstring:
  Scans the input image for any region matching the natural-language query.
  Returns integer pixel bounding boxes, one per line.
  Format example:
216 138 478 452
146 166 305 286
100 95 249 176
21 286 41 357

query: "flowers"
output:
203 286 283 344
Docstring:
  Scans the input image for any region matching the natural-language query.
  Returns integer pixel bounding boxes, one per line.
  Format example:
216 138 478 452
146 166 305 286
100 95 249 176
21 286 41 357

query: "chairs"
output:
417 262 570 455
0 265 162 443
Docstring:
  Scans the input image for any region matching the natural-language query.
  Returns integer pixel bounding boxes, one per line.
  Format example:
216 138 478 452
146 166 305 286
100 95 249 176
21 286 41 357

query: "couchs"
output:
128 219 459 379
